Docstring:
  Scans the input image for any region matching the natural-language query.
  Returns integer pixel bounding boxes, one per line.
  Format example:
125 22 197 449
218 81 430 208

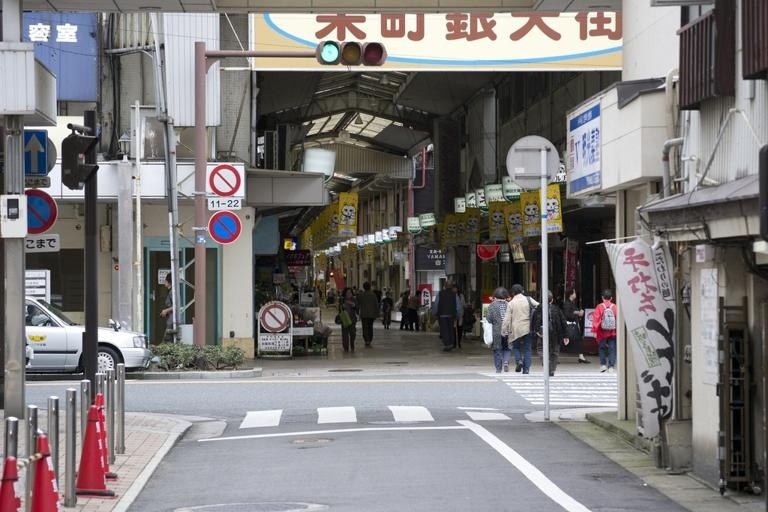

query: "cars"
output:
21 296 153 382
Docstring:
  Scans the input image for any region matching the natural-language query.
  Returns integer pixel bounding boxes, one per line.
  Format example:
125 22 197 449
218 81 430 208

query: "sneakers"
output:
600 365 607 372
495 360 554 377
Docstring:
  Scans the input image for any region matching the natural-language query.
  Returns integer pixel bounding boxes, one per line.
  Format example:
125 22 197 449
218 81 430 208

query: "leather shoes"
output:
578 358 591 363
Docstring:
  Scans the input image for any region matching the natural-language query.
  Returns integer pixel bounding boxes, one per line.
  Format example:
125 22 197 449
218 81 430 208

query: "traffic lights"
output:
60 133 98 190
314 39 389 66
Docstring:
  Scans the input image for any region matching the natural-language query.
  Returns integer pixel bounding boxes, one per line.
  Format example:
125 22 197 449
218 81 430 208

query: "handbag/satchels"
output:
335 302 344 324
540 319 554 336
565 320 582 342
338 310 353 329
527 297 536 338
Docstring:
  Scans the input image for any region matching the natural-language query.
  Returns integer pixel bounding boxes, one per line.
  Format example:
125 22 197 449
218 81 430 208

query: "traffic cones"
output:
0 392 118 511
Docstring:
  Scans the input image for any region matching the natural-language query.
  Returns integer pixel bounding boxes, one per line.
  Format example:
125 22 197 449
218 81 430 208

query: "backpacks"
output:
600 303 616 331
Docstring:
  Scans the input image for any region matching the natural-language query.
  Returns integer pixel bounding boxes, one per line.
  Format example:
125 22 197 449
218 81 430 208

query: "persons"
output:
27 303 52 326
290 279 617 377
158 272 171 344
